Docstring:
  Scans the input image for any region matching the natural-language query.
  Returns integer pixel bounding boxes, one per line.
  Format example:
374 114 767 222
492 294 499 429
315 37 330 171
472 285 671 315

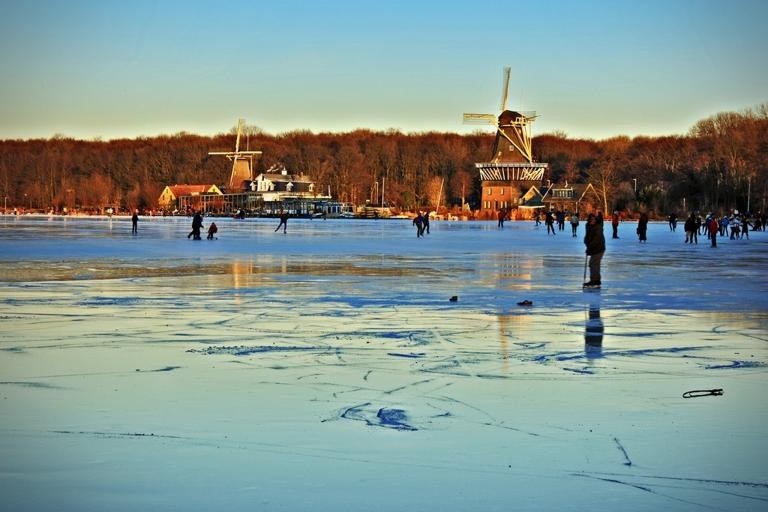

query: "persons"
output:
236 208 241 214
412 213 424 237
583 215 604 287
193 213 204 239
637 211 648 242
422 212 431 233
207 222 217 239
611 210 619 238
242 208 246 214
596 211 603 229
188 211 204 240
132 211 137 233
668 209 768 247
584 213 594 246
498 210 507 227
275 212 290 233
533 208 580 238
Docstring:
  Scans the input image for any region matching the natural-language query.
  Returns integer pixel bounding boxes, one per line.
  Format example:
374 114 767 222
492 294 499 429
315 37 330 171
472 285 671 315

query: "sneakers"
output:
582 279 601 287
